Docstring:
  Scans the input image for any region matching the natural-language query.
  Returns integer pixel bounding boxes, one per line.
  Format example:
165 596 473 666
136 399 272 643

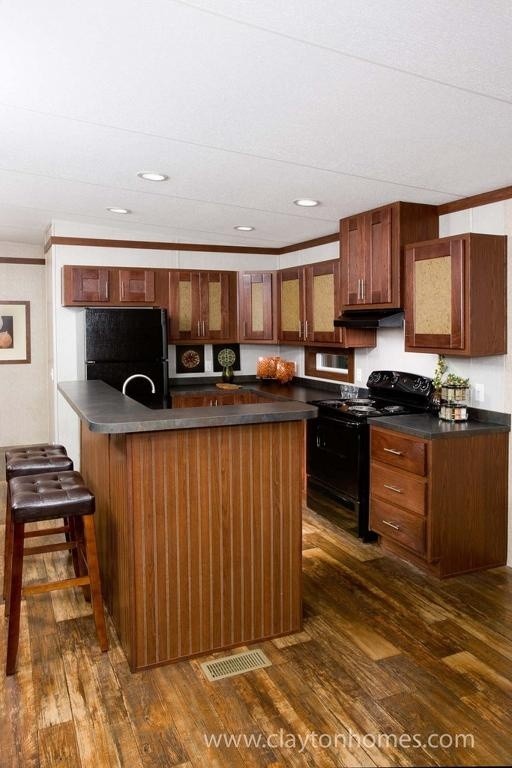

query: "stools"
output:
2 470 110 677
3 442 93 618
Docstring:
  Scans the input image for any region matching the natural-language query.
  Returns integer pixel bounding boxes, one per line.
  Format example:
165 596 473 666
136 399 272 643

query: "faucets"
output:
121 373 157 396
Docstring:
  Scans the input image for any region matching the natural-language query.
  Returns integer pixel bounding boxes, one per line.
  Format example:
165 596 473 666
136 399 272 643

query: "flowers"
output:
431 355 470 390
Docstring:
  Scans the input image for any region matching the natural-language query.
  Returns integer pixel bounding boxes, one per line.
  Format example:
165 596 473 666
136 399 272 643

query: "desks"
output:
53 378 323 677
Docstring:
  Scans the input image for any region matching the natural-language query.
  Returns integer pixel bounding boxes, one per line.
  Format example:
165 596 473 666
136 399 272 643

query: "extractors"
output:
335 309 405 330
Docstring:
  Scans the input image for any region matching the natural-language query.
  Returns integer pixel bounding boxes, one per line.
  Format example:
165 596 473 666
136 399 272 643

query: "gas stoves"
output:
317 396 426 418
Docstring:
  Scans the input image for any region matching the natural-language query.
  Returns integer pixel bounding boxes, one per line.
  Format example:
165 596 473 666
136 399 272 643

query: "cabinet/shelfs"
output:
366 420 509 579
239 269 275 343
171 392 238 410
236 387 275 405
404 231 509 358
61 264 163 307
275 259 377 350
337 200 440 311
164 267 239 345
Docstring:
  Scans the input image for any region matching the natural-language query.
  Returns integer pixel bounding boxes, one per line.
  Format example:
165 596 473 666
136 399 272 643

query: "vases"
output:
438 387 470 423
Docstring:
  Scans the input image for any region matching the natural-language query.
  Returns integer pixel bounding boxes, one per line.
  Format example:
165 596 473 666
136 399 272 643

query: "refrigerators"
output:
76 307 171 408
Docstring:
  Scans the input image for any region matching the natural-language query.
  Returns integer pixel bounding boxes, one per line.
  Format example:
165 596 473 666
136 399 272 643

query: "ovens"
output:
307 414 368 539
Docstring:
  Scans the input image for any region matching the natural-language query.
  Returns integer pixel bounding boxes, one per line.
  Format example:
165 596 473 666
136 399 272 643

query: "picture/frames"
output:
0 300 31 364
212 343 240 372
175 345 205 374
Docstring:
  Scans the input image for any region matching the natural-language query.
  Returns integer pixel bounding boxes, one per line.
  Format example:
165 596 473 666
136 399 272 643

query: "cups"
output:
337 385 359 398
